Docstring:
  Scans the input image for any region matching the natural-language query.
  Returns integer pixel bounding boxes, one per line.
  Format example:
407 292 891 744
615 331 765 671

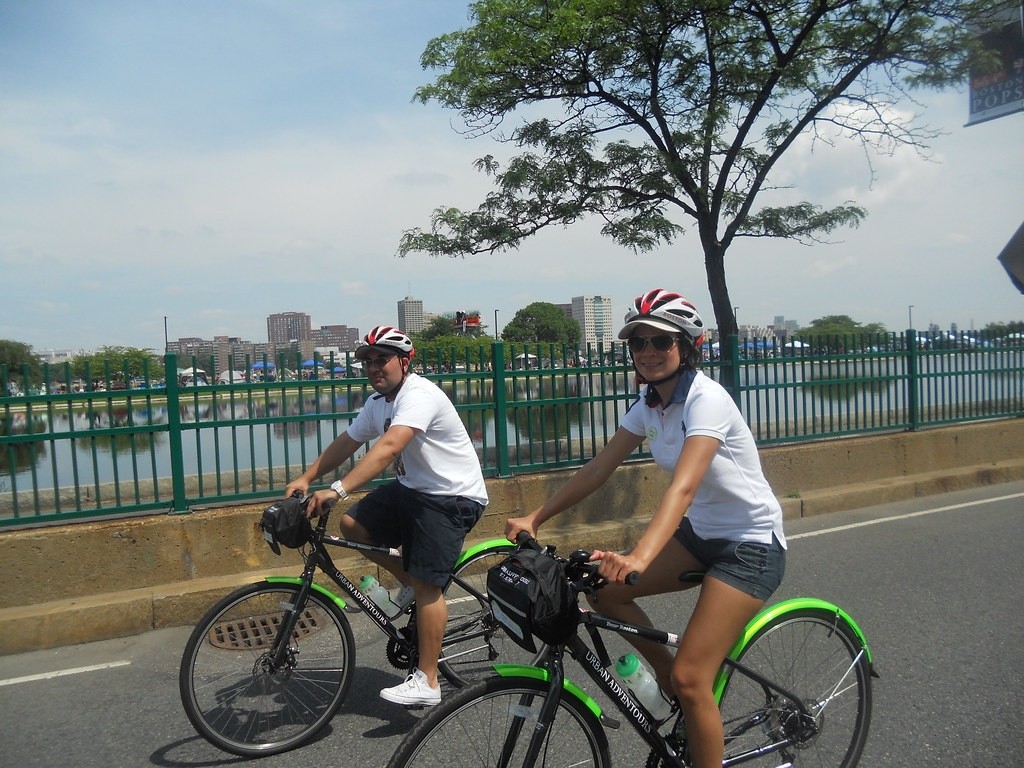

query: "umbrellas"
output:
516 353 537 359
334 366 346 373
349 362 363 369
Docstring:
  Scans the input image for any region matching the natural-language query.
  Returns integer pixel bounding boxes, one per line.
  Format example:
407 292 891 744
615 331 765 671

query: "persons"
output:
285 326 488 705
503 290 787 768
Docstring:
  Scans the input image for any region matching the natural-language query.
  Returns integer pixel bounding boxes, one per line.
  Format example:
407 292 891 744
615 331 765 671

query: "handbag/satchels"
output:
487 549 580 653
259 497 312 556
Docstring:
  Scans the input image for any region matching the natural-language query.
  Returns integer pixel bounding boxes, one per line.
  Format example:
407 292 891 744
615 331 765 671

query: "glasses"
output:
626 335 680 354
361 355 397 372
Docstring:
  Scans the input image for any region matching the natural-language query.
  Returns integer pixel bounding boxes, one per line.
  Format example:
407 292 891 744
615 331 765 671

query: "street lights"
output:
494 310 499 341
734 306 739 321
909 305 913 329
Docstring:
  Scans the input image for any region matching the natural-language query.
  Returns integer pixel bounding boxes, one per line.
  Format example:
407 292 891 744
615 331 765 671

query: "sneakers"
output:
380 667 442 704
385 586 415 622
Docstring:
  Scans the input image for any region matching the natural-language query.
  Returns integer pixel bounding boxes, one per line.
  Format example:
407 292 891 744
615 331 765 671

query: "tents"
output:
251 362 276 378
703 341 809 359
179 366 206 385
301 359 324 369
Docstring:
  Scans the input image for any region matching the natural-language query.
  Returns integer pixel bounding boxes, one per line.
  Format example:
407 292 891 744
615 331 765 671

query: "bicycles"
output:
178 490 554 757
387 528 881 768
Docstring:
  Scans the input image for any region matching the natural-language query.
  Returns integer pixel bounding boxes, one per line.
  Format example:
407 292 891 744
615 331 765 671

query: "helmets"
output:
360 326 415 362
624 288 704 352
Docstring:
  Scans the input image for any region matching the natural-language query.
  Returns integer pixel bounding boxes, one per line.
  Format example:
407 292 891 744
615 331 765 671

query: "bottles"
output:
615 653 672 721
358 575 401 619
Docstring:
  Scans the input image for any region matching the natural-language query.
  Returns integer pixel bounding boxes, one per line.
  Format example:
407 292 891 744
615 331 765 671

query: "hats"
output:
355 345 410 362
618 318 683 340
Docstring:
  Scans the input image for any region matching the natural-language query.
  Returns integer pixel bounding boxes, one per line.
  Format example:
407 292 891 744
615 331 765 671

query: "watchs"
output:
331 480 349 501
